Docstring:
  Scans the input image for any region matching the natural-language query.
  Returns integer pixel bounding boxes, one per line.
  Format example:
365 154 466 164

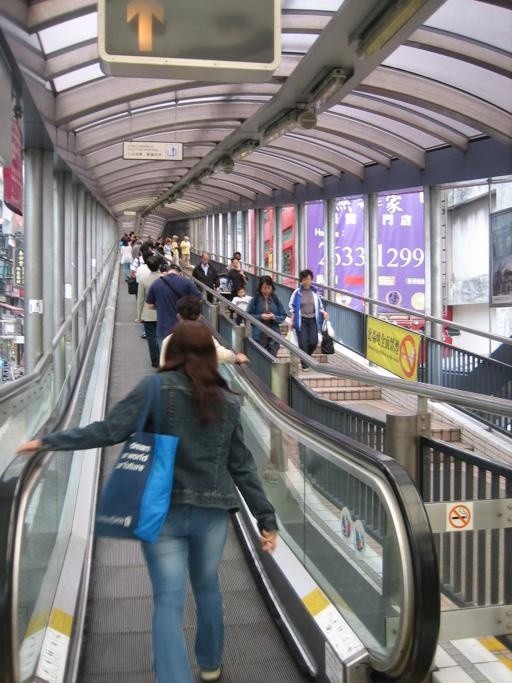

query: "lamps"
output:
197 170 213 182
160 199 167 208
357 0 425 59
310 69 345 110
230 139 260 161
168 192 178 204
212 159 233 173
180 185 188 193
263 109 308 142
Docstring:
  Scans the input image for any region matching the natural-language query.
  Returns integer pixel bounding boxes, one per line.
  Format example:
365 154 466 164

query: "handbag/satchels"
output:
125 277 138 295
320 318 336 355
92 373 181 546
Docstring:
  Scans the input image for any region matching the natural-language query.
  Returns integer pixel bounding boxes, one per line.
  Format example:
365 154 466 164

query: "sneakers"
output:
301 366 310 373
199 663 222 683
